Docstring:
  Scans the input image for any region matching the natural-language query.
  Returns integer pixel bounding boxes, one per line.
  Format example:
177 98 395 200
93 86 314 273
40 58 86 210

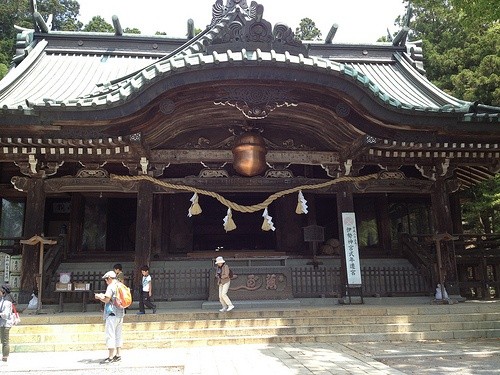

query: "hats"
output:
102 271 116 279
215 257 226 264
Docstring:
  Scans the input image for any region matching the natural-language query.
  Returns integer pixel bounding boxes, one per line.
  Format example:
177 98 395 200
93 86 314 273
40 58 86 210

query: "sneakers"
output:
219 308 227 312
227 305 235 312
100 356 121 365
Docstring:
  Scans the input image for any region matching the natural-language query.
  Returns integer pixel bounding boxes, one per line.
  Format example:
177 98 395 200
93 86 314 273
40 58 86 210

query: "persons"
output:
95 270 125 363
0 284 14 362
215 257 235 311
136 264 156 314
113 264 124 285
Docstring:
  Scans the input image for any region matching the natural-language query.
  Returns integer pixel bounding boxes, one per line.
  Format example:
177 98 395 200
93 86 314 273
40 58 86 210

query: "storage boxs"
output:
74 282 90 290
56 282 68 291
68 283 72 290
60 273 72 282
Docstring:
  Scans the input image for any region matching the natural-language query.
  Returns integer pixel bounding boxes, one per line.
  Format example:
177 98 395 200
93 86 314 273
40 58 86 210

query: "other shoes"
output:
153 306 157 314
0 358 7 365
137 311 146 315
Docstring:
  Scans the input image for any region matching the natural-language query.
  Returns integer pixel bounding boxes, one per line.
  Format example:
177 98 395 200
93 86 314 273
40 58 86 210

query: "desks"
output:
54 290 91 313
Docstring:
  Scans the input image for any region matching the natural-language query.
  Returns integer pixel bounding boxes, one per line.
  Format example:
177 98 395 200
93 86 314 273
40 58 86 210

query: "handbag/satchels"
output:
4 301 20 325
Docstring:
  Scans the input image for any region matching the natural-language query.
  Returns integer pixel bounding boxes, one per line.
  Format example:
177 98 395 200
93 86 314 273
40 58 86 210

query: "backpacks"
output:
110 282 132 309
228 268 233 279
